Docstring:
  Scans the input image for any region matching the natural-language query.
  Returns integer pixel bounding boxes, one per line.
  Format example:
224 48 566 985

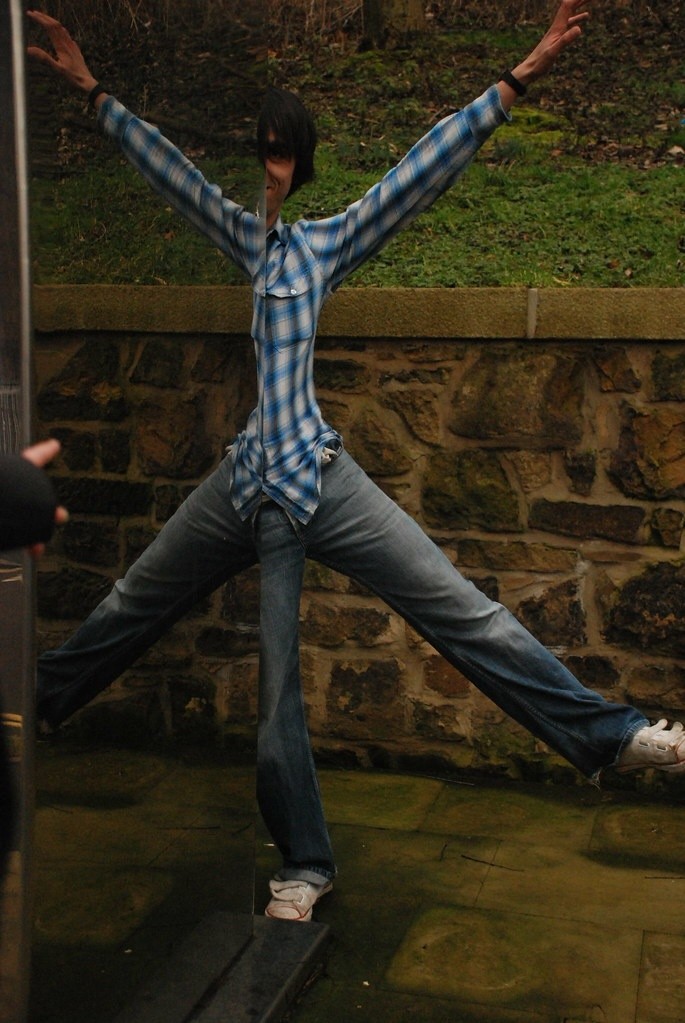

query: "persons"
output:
27 10 259 742
259 0 685 920
0 438 70 556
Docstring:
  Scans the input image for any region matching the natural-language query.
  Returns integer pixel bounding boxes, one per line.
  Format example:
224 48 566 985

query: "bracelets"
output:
88 84 104 105
498 68 527 97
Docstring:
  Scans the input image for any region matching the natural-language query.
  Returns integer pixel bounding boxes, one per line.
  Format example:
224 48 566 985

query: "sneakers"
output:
266 868 333 922
618 719 685 774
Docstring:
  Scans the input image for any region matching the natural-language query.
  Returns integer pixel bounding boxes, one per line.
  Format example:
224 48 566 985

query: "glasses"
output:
267 139 299 165
